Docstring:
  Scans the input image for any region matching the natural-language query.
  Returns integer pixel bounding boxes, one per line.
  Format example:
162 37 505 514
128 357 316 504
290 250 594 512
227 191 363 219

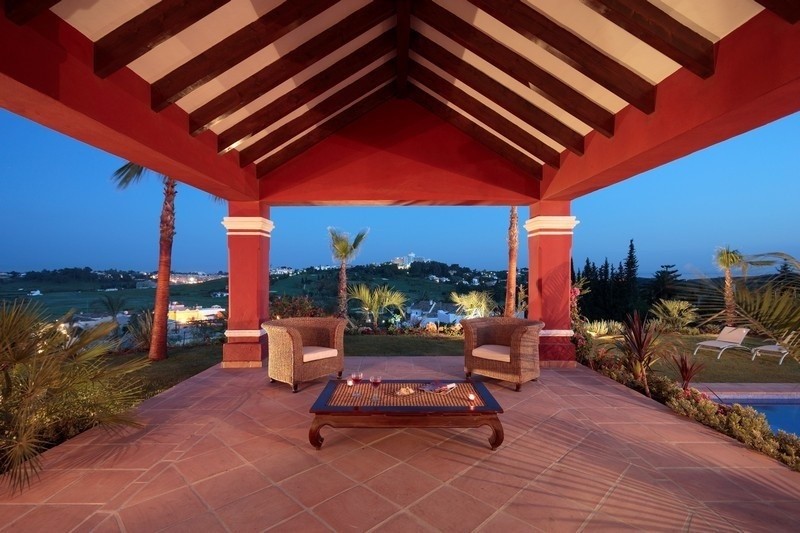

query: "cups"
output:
468 391 476 401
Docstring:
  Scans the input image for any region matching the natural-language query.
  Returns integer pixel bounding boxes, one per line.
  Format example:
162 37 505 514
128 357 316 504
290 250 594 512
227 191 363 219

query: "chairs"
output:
692 326 752 360
750 331 800 365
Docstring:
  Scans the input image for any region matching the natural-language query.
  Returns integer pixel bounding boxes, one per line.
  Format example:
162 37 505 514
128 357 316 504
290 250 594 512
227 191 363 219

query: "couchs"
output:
261 317 349 391
460 317 545 393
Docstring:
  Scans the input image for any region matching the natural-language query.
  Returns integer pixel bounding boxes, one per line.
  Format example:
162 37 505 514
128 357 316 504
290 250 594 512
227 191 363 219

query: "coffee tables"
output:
308 377 506 452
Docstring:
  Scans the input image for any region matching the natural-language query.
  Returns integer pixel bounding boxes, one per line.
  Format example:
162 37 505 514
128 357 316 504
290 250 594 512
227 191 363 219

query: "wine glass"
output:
369 375 382 402
351 372 363 397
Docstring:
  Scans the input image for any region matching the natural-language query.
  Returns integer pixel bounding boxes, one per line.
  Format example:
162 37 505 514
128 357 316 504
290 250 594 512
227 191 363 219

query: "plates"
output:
419 383 457 395
